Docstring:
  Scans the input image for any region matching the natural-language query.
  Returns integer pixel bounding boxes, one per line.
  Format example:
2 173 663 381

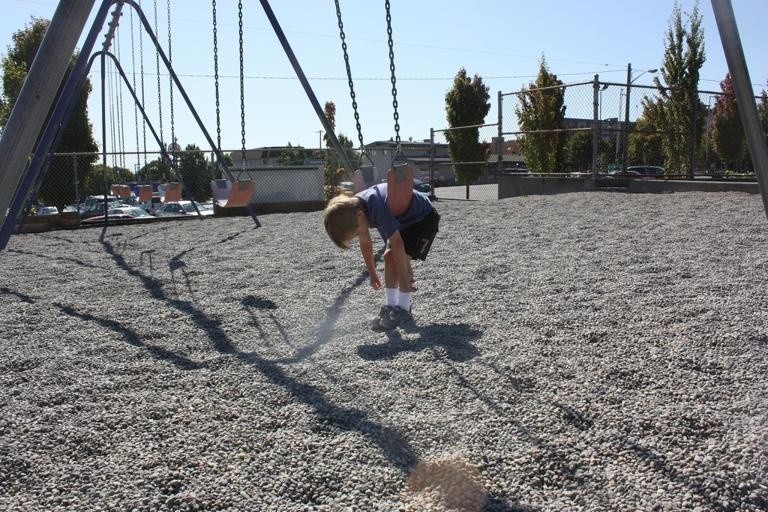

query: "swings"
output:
153 1 185 204
207 0 256 212
129 0 154 203
336 0 413 220
101 12 132 200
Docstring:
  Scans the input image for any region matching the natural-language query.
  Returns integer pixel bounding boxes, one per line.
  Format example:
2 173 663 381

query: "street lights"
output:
134 163 141 182
704 95 720 175
623 68 659 177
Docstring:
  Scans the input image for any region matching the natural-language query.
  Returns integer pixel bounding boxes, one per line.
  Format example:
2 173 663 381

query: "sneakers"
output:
371 305 412 330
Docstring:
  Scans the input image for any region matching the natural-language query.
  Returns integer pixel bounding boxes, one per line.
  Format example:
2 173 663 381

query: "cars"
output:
711 171 723 178
338 181 353 193
5 190 218 225
599 170 645 181
412 176 431 193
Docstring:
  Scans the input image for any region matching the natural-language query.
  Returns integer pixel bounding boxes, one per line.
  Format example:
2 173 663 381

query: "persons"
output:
322 180 441 331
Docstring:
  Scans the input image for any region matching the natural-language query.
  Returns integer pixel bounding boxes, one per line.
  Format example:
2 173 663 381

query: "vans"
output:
626 166 665 181
